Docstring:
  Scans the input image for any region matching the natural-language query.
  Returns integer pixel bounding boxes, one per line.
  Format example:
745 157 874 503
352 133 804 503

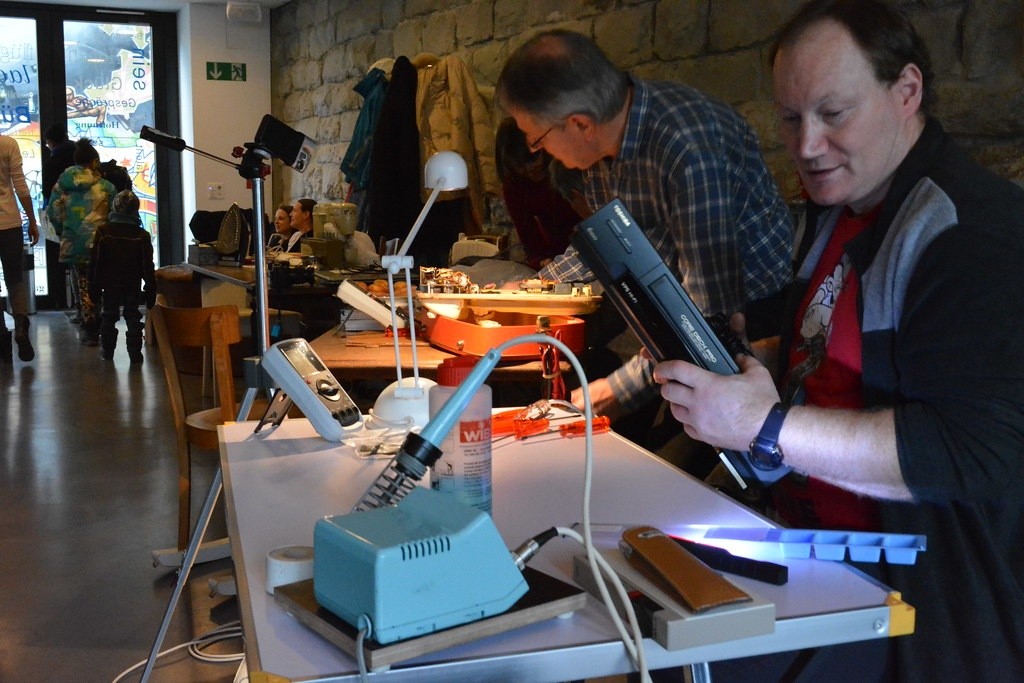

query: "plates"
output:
332 293 407 303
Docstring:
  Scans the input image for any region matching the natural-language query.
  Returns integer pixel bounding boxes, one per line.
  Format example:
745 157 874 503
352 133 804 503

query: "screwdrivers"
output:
492 417 549 443
522 415 611 441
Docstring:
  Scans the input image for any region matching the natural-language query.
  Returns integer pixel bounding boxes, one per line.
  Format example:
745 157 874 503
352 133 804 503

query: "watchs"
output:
748 400 790 473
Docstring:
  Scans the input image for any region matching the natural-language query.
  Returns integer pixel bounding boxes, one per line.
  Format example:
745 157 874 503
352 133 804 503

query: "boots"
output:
14 314 34 362
0 330 13 365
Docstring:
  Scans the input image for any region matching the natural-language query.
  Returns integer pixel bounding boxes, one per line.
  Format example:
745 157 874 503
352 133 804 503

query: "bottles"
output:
429 355 492 518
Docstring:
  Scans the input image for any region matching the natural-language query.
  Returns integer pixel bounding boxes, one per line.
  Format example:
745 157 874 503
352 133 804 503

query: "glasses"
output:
524 111 592 151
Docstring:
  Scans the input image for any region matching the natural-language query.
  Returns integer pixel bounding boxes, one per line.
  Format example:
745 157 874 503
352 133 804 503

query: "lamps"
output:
381 150 469 397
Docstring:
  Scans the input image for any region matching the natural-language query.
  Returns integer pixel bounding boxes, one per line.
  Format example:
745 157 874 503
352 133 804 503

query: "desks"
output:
308 325 571 380
214 407 915 683
182 261 420 290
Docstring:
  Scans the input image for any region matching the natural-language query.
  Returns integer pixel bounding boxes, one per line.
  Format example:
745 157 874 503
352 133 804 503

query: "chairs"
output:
451 232 510 265
149 294 306 551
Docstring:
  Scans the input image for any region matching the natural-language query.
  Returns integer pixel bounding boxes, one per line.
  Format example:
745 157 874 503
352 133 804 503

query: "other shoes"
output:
82 334 99 346
130 352 142 364
102 350 114 360
69 315 84 322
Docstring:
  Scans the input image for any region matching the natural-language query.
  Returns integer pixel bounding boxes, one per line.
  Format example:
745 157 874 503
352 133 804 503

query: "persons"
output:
0 131 318 369
655 1 1024 683
494 32 796 513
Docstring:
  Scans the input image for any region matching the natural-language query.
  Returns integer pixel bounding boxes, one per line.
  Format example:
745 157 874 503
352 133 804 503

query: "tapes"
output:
264 544 314 595
